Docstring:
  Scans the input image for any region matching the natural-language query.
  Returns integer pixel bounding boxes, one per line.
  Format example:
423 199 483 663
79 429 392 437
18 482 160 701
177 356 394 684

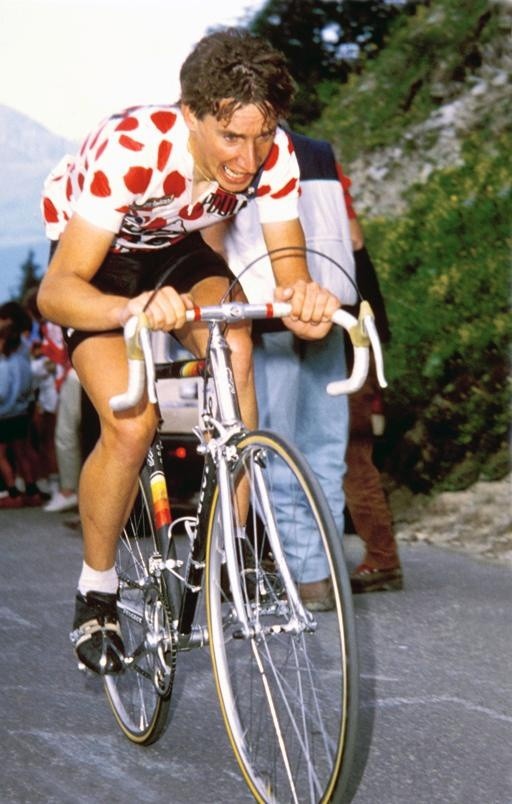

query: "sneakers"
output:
218 540 283 604
70 587 127 676
21 489 45 507
277 579 336 614
0 492 25 510
42 485 79 513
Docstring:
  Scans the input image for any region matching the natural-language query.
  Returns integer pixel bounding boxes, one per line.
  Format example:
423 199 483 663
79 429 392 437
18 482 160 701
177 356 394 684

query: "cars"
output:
127 328 206 536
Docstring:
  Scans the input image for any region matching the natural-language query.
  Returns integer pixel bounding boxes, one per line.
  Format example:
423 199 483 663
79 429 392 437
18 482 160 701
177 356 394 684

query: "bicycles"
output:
78 247 388 804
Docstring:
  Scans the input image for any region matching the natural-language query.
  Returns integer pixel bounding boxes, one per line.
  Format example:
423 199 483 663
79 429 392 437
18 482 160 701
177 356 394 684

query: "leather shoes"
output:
348 561 404 592
